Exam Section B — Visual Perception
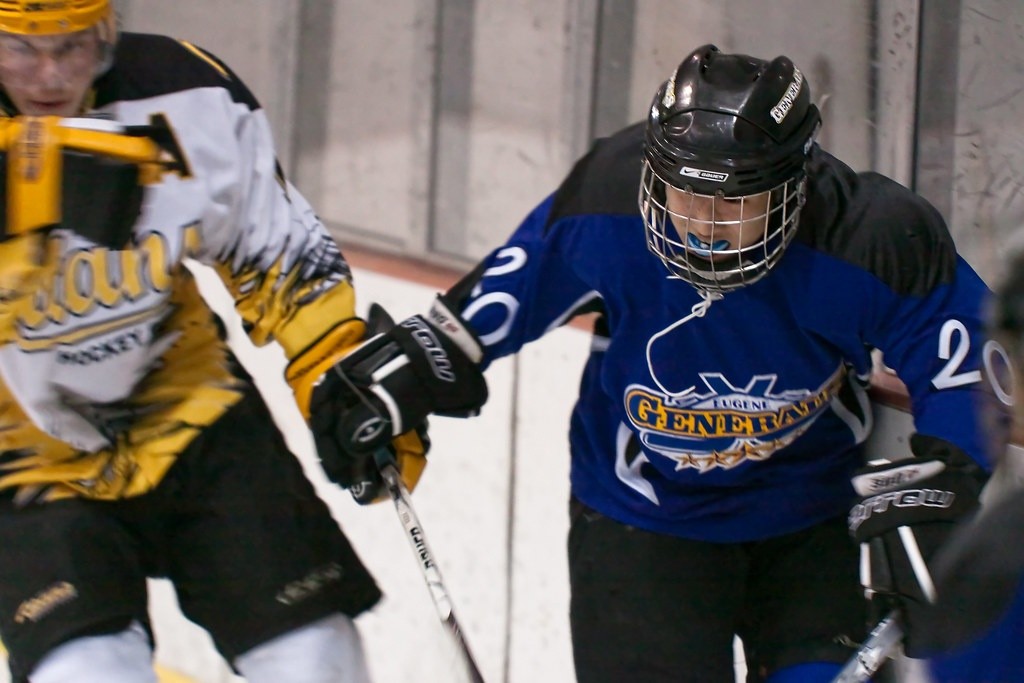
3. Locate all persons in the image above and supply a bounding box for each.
[311,43,1024,683]
[0,0,428,683]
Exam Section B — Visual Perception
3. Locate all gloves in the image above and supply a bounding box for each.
[308,292,490,506]
[282,317,428,504]
[840,452,980,661]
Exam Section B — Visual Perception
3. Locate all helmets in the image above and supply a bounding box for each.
[638,44,823,293]
[0,0,117,46]
